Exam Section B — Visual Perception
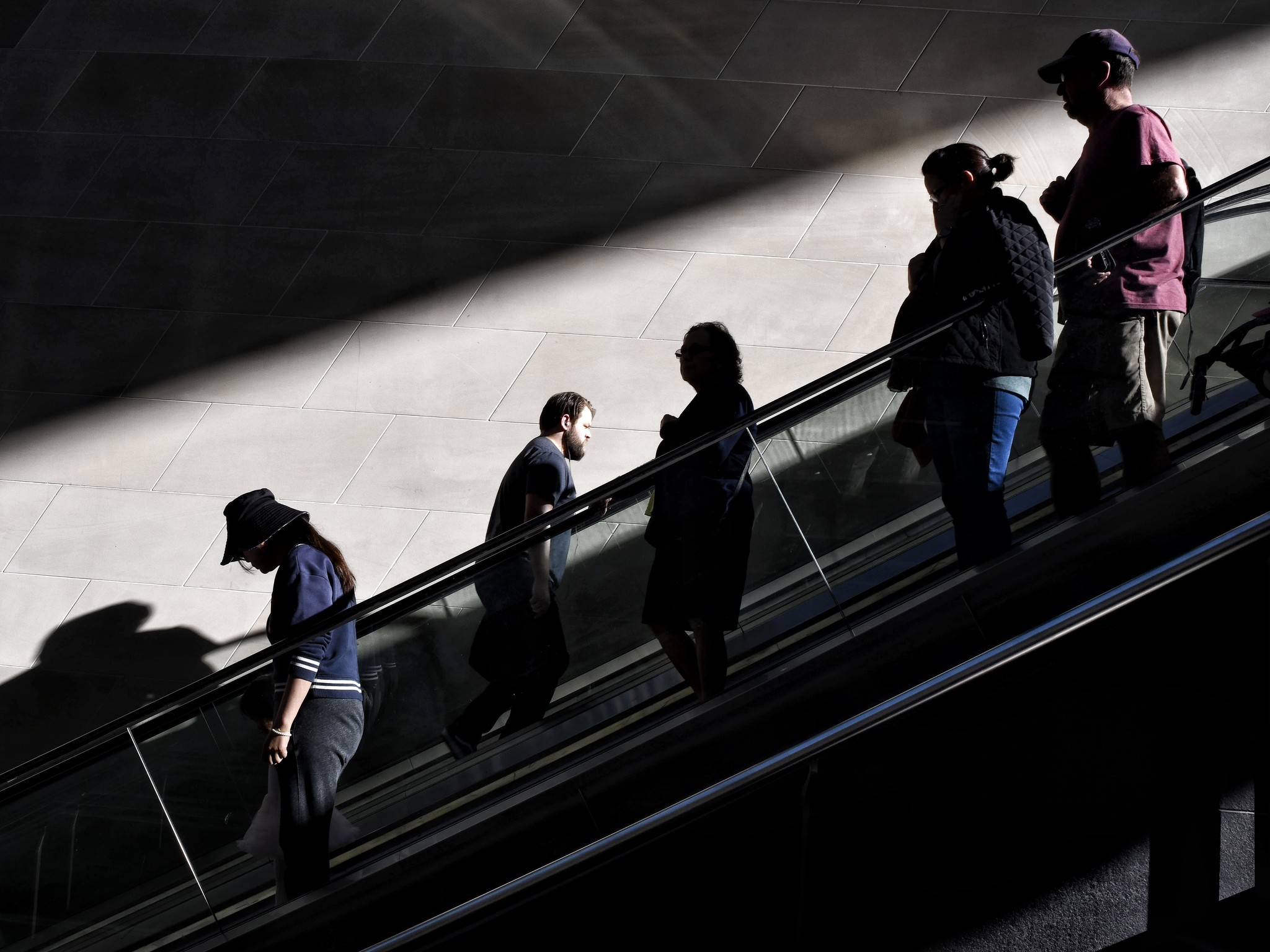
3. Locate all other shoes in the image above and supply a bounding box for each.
[441,724,477,761]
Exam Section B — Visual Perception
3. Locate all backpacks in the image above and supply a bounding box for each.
[1110,105,1204,317]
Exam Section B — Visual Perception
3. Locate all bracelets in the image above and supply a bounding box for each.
[276,727,291,733]
[270,727,292,736]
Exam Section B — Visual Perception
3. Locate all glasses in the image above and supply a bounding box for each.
[674,349,712,359]
[929,182,950,203]
[1059,69,1097,82]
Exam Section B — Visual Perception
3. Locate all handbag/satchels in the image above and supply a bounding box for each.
[236,762,358,858]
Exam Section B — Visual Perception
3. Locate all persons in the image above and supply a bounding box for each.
[641,321,757,706]
[887,144,1055,572]
[219,488,365,908]
[1038,29,1189,521]
[442,391,612,760]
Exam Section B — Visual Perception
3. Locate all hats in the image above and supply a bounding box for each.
[220,488,310,566]
[1037,29,1139,84]
